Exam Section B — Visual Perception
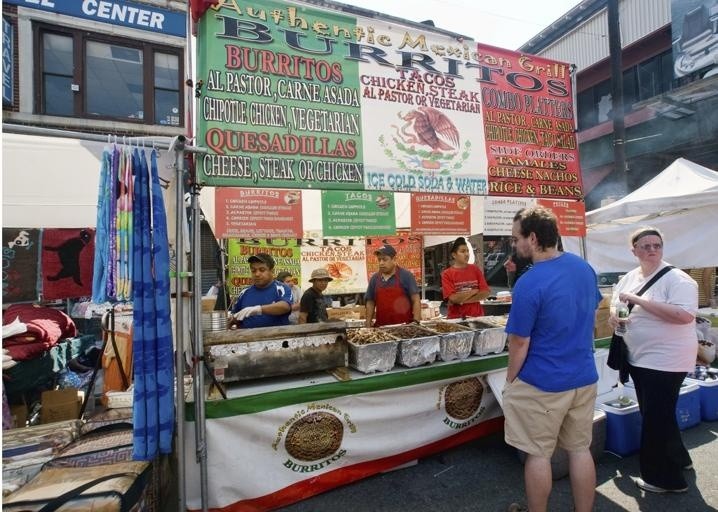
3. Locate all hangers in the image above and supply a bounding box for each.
[96,134,164,157]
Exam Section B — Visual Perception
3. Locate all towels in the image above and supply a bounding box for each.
[87,146,176,462]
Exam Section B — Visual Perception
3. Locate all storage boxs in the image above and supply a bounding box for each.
[697,369,717,423]
[624,377,703,433]
[519,407,608,479]
[597,393,645,459]
[8,393,28,428]
[41,386,84,423]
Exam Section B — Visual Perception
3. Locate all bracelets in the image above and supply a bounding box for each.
[412,320,419,325]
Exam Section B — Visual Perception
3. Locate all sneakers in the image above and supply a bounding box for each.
[636,478,688,494]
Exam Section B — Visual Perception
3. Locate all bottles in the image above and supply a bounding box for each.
[614,304,628,335]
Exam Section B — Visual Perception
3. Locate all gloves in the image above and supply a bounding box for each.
[233,305,262,321]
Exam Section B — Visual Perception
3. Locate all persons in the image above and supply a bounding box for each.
[297,269,333,323]
[363,246,421,327]
[277,272,295,291]
[440,238,491,319]
[228,252,294,329]
[502,255,517,290]
[609,225,702,495]
[501,203,602,511]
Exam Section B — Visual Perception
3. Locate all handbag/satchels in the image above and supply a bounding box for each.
[607,330,629,386]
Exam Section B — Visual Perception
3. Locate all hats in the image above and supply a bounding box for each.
[448,237,465,252]
[308,269,333,282]
[248,252,274,267]
[373,245,395,257]
